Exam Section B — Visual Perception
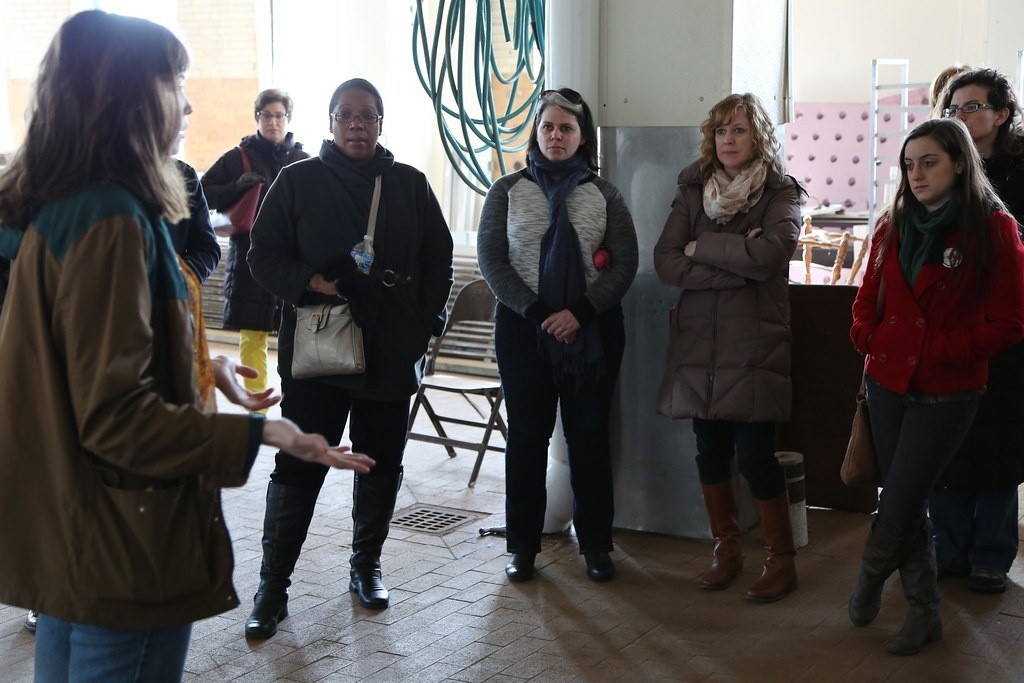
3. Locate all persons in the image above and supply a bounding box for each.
[654,94,798,600]
[246,79,454,638]
[849,119,1024,655]
[929,65,1024,594]
[478,88,639,581]
[199,90,309,415]
[0,10,376,683]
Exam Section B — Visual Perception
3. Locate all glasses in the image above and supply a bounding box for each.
[257,111,288,121]
[539,88,586,111]
[331,111,383,124]
[942,102,995,118]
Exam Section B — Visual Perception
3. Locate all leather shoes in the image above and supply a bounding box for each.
[583,552,614,580]
[506,552,536,581]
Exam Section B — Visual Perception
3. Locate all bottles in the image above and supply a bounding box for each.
[336,235,376,301]
[593,248,612,270]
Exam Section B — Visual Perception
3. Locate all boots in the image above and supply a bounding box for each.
[244,481,320,640]
[885,519,945,651]
[849,513,925,628]
[696,483,743,589]
[745,493,797,602]
[350,465,404,608]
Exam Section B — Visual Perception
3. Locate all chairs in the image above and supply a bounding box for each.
[407,279,508,491]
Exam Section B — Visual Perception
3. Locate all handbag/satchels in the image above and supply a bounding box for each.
[291,304,365,380]
[211,146,262,237]
[840,400,884,486]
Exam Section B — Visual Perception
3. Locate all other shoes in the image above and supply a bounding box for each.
[972,565,1007,592]
[936,555,958,577]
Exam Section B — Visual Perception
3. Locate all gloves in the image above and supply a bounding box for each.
[235,172,266,195]
